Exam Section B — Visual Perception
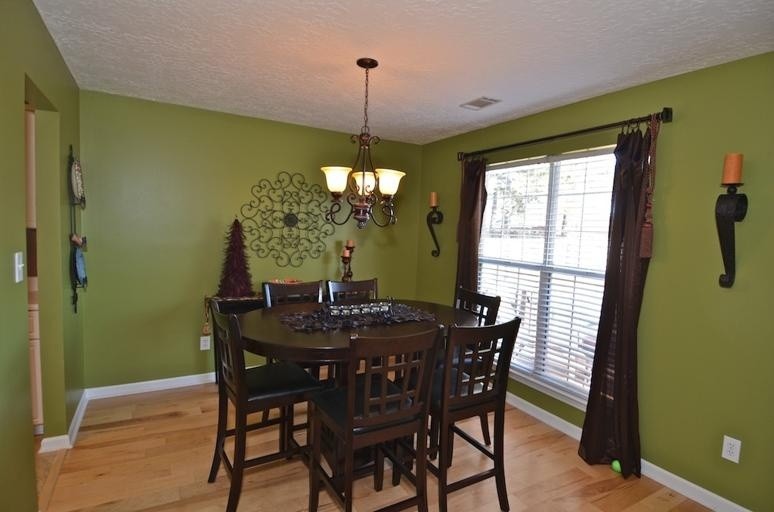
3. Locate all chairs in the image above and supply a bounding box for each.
[309,324,448,512]
[209,299,323,512]
[397,286,503,461]
[258,279,327,453]
[392,316,524,512]
[328,277,379,304]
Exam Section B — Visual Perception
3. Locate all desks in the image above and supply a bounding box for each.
[237,299,479,482]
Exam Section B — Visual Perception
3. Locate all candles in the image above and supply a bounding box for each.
[722,153,743,184]
[429,191,438,207]
[343,240,353,257]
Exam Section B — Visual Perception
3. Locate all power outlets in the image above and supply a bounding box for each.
[721,435,742,464]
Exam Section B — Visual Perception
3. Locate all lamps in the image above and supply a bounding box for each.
[320,57,407,230]
